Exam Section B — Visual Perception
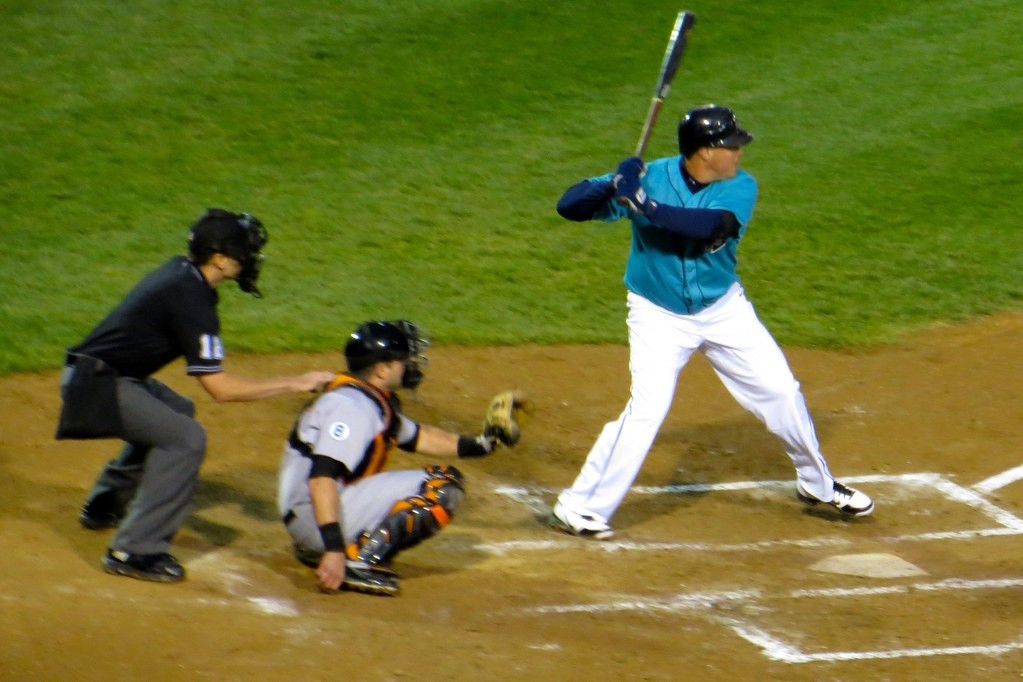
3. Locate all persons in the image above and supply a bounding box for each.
[278,320,534,596]
[549,104,875,540]
[55,207,334,584]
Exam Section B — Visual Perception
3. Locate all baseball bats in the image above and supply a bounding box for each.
[616,10,697,208]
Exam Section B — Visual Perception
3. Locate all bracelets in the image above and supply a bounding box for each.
[320,522,345,552]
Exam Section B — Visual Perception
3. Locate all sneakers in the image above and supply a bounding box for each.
[550,499,614,541]
[79,503,129,529]
[795,474,873,518]
[101,546,183,582]
[340,561,399,596]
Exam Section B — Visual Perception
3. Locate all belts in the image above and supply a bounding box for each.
[282,510,296,527]
[66,353,79,366]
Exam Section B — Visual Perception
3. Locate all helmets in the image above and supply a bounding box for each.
[188,205,267,266]
[677,103,753,159]
[344,320,408,371]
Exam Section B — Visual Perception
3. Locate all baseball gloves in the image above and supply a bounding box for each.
[482,388,535,450]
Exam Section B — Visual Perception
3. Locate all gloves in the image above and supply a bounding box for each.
[616,178,656,218]
[613,156,648,187]
